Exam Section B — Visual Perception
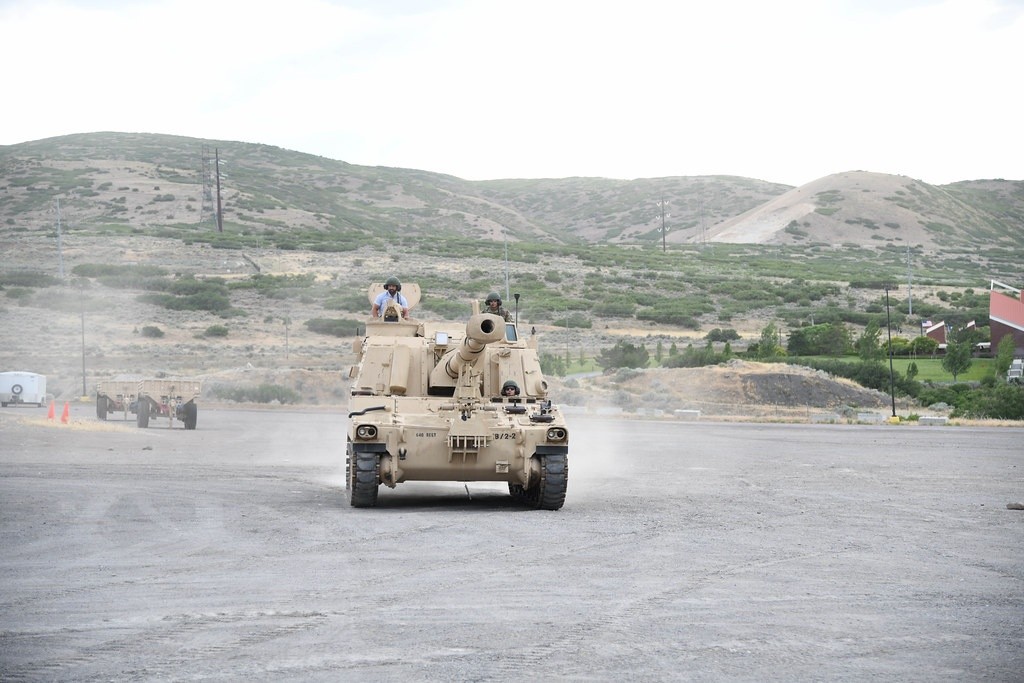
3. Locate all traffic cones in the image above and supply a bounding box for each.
[59,401,69,422]
[46,401,56,420]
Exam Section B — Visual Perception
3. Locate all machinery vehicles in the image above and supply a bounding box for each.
[347,282,570,511]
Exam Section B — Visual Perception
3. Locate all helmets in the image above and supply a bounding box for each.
[383,276,401,291]
[485,293,502,306]
[501,380,520,394]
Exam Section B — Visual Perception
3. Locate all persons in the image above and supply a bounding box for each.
[482,293,515,323]
[501,380,521,397]
[371,276,409,320]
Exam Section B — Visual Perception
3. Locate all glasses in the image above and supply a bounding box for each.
[490,300,497,302]
[506,388,514,390]
